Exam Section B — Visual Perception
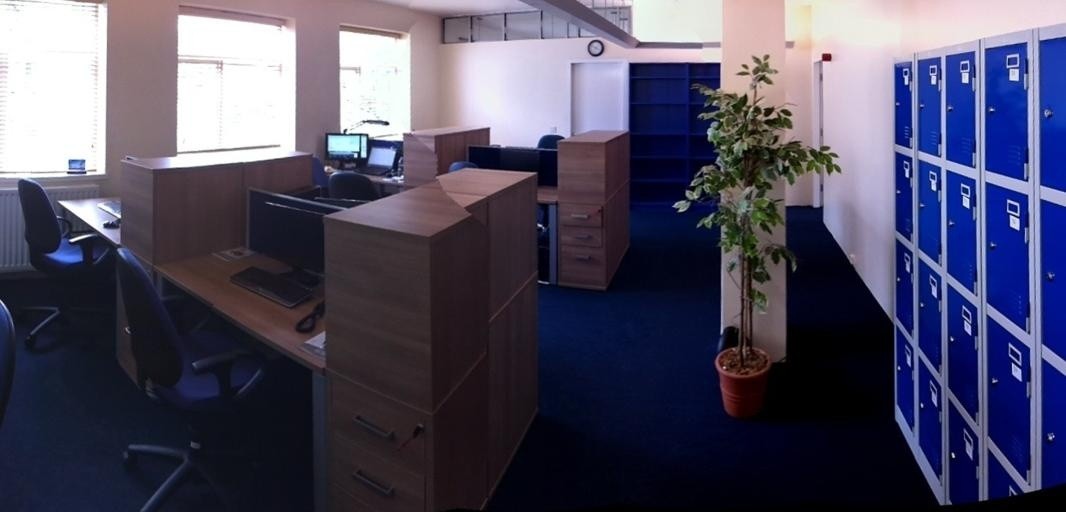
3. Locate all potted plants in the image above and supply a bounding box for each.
[672,54,843,418]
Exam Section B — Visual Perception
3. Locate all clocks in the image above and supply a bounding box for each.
[587,40,604,57]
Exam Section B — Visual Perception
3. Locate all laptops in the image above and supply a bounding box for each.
[353,147,397,175]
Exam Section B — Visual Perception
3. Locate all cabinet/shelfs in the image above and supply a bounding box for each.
[628,62,720,205]
[893,23,1066,512]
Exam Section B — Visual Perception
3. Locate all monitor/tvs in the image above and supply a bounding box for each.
[325,132,369,171]
[245,186,348,286]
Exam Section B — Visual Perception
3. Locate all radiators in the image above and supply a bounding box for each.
[0,184,101,272]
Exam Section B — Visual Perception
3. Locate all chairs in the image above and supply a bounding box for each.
[16,179,110,347]
[0,301,17,416]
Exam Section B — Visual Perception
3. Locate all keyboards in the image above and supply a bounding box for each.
[98,199,121,219]
[231,267,312,307]
